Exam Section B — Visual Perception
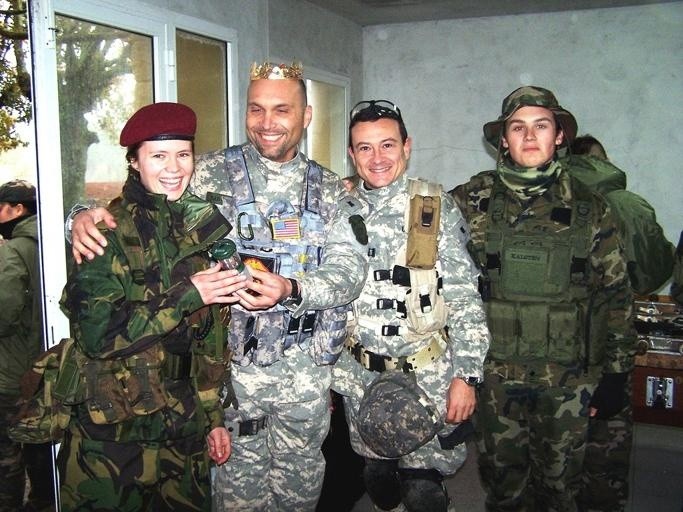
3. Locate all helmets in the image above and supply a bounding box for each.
[357,370,444,458]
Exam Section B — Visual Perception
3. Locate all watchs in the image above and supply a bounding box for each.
[280,277,299,307]
[461,376,481,385]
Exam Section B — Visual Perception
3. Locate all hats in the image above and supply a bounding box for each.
[0,180,35,196]
[483,86,577,152]
[120,102,196,147]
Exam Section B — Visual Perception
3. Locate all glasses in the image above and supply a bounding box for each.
[349,100,401,122]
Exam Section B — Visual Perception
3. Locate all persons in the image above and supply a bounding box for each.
[445,87,638,512]
[64,67,370,511]
[328,107,495,512]
[56,102,232,512]
[560,134,677,512]
[0,179,58,512]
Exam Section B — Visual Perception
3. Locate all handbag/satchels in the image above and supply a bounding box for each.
[8,337,78,445]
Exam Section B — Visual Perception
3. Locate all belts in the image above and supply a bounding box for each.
[345,331,449,373]
[287,313,317,335]
